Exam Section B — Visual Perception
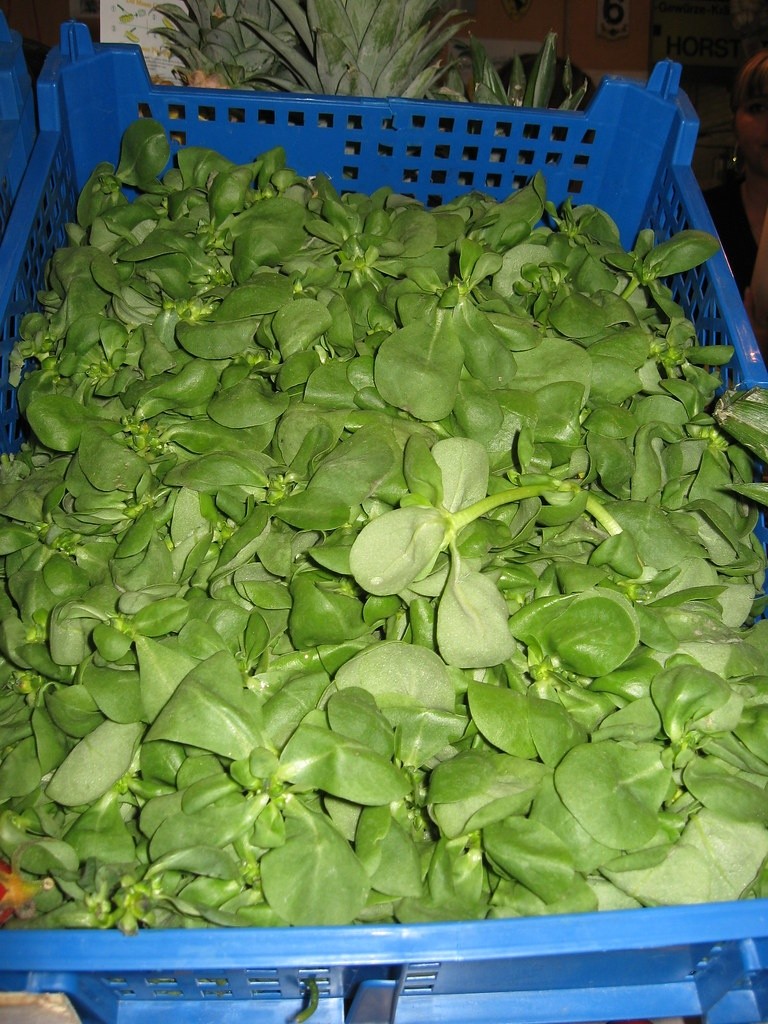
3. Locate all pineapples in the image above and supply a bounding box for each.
[146,0,311,145]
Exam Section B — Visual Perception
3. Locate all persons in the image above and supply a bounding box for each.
[702,46,768,363]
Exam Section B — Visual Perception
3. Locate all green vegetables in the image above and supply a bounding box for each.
[0,109,767,934]
